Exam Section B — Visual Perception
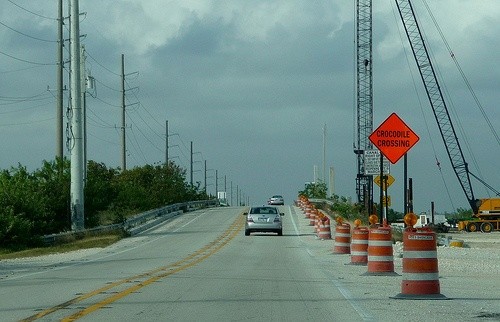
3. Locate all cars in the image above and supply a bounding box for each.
[267,198,271,205]
[243,205,285,236]
[269,194,284,205]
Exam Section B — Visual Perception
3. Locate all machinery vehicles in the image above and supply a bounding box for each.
[457,198,500,233]
